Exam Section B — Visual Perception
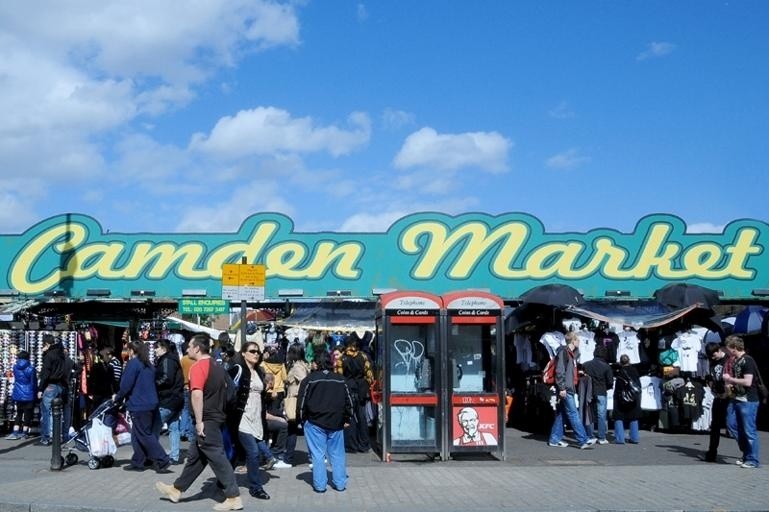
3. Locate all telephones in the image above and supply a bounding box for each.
[416,357,432,389]
[449,358,463,388]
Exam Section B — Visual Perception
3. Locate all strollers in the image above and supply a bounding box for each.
[51,395,126,470]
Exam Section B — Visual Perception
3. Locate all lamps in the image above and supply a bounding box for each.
[716,290,725,297]
[577,289,585,297]
[278,289,304,297]
[1,290,20,297]
[87,289,111,299]
[131,290,156,297]
[327,290,352,296]
[182,289,207,297]
[373,289,397,296]
[45,288,70,296]
[751,290,769,297]
[606,290,631,296]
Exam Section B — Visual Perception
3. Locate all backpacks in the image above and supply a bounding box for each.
[543,360,555,384]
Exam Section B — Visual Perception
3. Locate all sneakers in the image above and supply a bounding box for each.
[736,459,754,468]
[255,489,270,501]
[239,456,293,472]
[549,438,608,449]
[157,458,178,473]
[155,482,181,502]
[34,442,48,446]
[5,433,18,440]
[213,496,243,511]
[124,465,144,471]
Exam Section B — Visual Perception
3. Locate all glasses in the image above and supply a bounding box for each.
[248,350,261,354]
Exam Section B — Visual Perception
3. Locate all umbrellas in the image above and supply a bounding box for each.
[653,282,719,322]
[731,305,769,336]
[520,283,586,324]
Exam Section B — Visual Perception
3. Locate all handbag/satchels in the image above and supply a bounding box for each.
[622,379,640,403]
